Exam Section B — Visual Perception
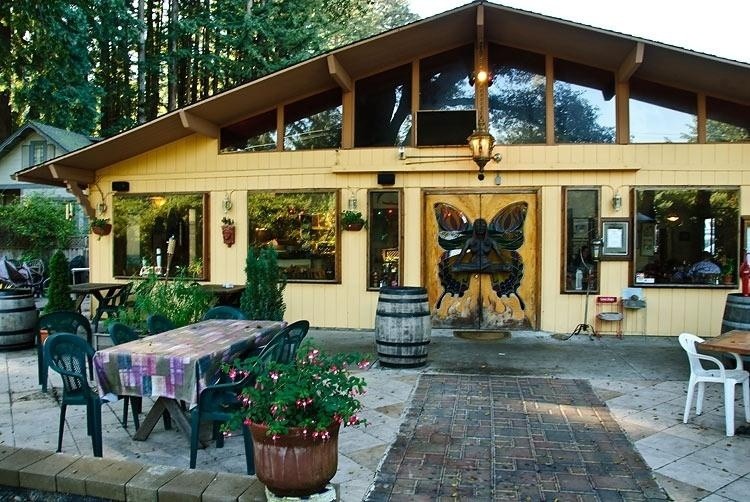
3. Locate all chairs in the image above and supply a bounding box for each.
[185,320,309,476]
[679,332,750,437]
[44,305,245,460]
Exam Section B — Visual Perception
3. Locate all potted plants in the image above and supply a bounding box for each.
[91,217,112,235]
[339,210,365,231]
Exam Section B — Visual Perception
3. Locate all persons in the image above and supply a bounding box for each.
[451,218,514,275]
[685,251,723,280]
[574,245,595,283]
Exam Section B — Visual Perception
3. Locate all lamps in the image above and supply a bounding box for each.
[613,189,622,212]
[466,115,502,173]
[348,192,357,210]
[222,191,232,215]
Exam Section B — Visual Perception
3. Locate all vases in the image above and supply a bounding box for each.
[248,424,340,498]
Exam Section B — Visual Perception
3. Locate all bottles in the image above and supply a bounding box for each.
[576,267,582,290]
[715,276,719,285]
[739,253,750,297]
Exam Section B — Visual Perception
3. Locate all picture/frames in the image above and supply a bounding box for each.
[600,217,634,261]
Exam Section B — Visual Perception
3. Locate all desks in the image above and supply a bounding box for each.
[67,280,245,330]
[697,330,750,355]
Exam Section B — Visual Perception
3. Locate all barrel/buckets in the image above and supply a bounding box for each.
[721,293,750,384]
[0,288,37,351]
[375,286,432,368]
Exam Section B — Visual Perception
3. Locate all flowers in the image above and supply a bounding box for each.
[219,343,371,440]
